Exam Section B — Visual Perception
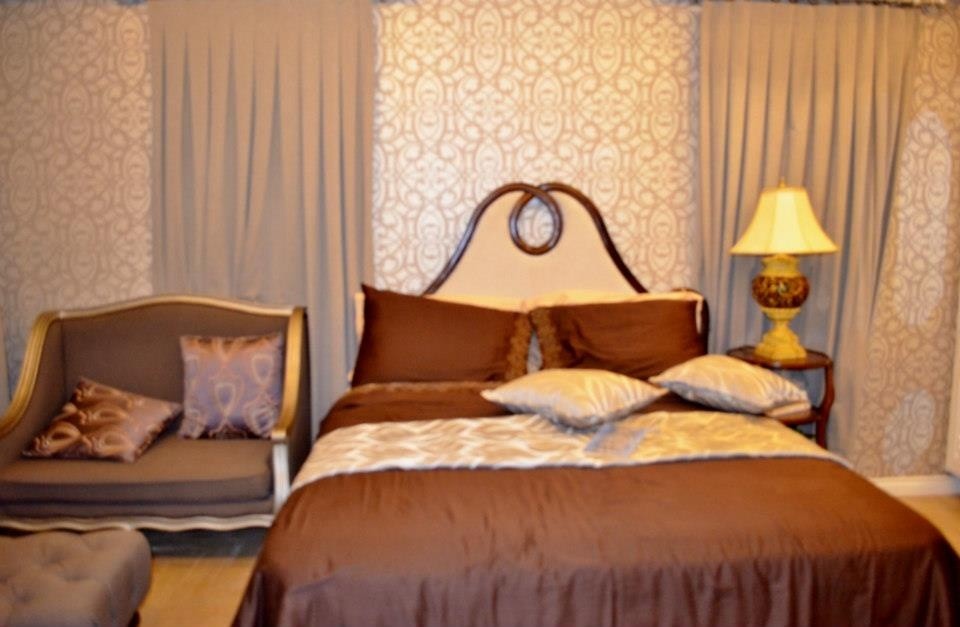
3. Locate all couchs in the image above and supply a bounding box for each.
[0,532,154,627]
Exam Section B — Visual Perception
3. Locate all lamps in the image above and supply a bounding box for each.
[729,175,839,359]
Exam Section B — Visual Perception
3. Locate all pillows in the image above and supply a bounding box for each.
[480,368,670,429]
[179,332,283,441]
[649,353,809,414]
[24,376,182,466]
[530,288,704,383]
[348,281,533,387]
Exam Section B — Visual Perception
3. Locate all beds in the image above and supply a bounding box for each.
[257,181,960,627]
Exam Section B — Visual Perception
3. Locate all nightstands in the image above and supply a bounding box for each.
[726,344,835,453]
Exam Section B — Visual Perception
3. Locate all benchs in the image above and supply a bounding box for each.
[0,297,315,532]
